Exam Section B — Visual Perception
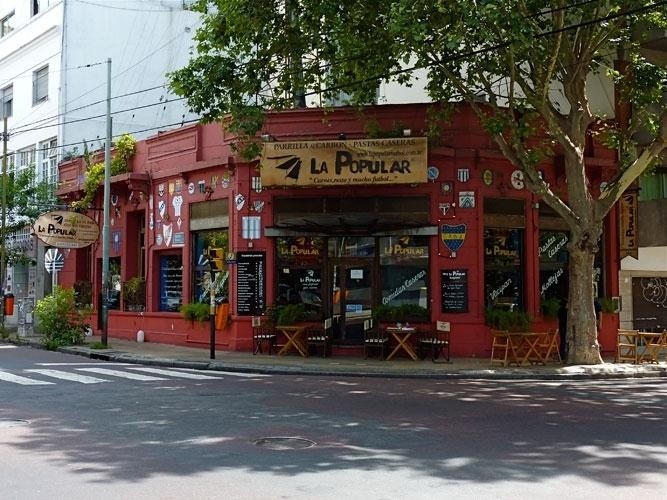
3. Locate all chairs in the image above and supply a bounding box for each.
[250,313,452,364]
[489,327,666,368]
